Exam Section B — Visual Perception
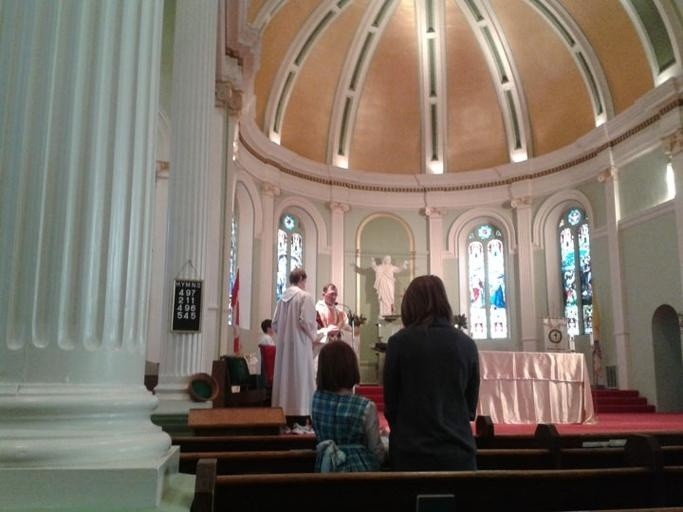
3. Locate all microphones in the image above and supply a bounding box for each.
[334,302,351,312]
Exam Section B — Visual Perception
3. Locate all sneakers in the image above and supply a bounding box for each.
[286,422,316,435]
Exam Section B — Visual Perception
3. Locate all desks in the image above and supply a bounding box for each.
[478,350,585,424]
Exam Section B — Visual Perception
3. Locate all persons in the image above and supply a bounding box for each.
[311,340,385,473]
[383,275,480,472]
[259,319,275,346]
[312,283,353,383]
[271,268,317,435]
[371,256,407,318]
